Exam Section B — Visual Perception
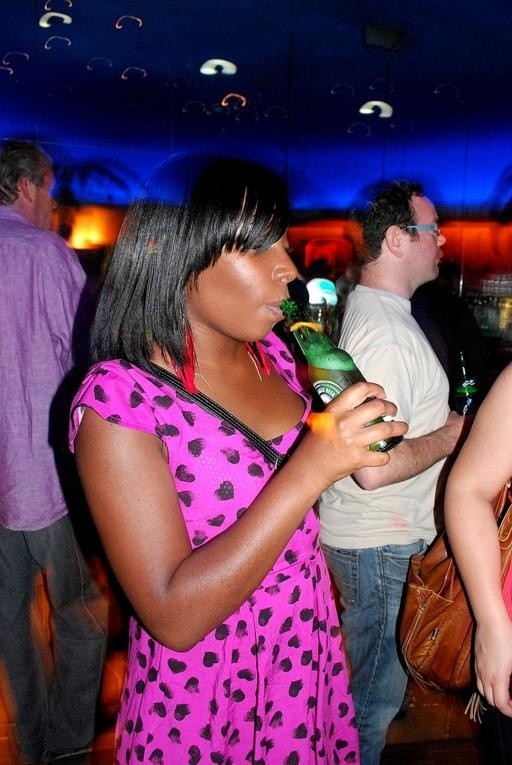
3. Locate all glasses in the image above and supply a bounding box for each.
[400,223,440,237]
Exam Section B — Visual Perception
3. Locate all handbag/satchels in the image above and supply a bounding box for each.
[396,478,512,697]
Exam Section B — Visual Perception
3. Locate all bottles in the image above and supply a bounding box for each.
[279,295,405,454]
[450,347,480,418]
